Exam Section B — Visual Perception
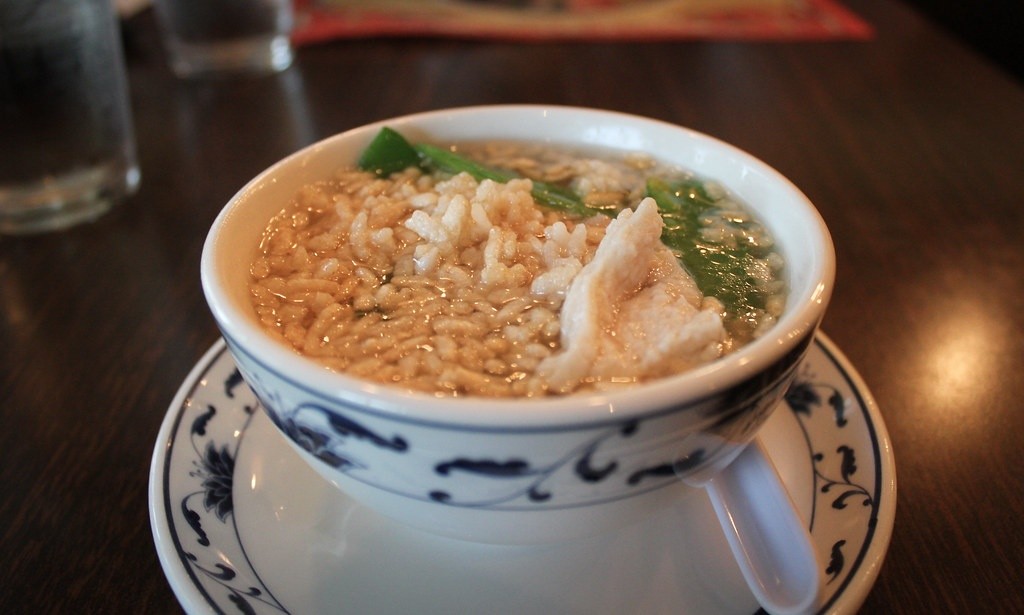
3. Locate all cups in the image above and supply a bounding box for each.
[0,0,146,237]
[154,0,294,79]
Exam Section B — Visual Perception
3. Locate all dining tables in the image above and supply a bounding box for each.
[0,0,1024,615]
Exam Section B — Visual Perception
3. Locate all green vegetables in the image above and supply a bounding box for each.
[355,124,785,322]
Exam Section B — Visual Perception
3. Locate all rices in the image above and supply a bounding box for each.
[250,141,783,399]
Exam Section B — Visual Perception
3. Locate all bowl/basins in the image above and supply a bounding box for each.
[200,104,836,546]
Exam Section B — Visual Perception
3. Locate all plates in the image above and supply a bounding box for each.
[148,324,898,615]
